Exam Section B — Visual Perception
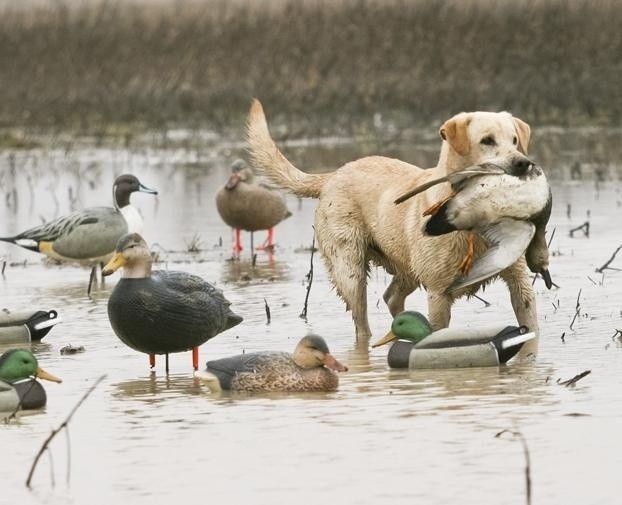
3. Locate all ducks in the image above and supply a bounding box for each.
[101,232,244,369]
[216,160,293,248]
[393,162,552,289]
[0,173,159,284]
[194,333,348,393]
[0,348,63,412]
[0,307,64,345]
[371,310,537,369]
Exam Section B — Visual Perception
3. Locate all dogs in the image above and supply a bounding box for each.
[242,97,540,339]
